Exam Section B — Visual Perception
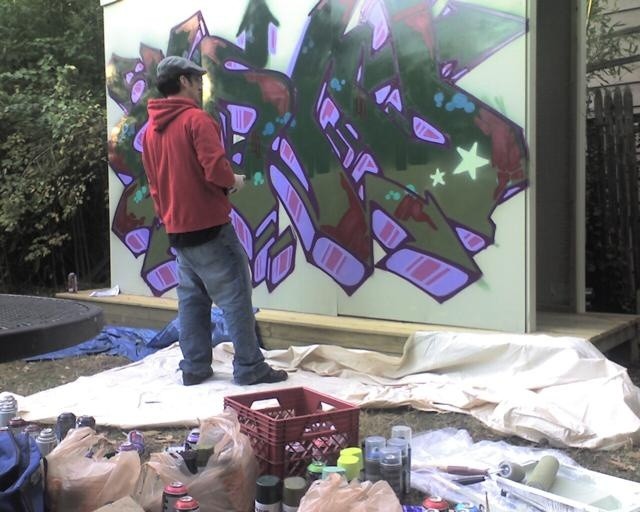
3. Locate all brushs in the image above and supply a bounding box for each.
[411,459,525,482]
[452,455,559,492]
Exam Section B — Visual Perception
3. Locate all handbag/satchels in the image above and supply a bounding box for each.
[0,428,46,512]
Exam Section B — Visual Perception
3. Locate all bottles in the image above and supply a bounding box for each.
[422,494,480,512]
[0,392,145,456]
[173,495,200,512]
[254,424,411,512]
[68,273,78,292]
[184,428,200,453]
[161,481,188,512]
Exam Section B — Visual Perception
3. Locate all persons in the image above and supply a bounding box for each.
[138,55,290,385]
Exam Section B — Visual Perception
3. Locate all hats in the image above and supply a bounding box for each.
[156,56,208,77]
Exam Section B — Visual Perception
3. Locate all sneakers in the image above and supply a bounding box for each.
[183,366,213,385]
[236,365,288,385]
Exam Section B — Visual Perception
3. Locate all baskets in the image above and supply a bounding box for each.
[224,386,360,476]
